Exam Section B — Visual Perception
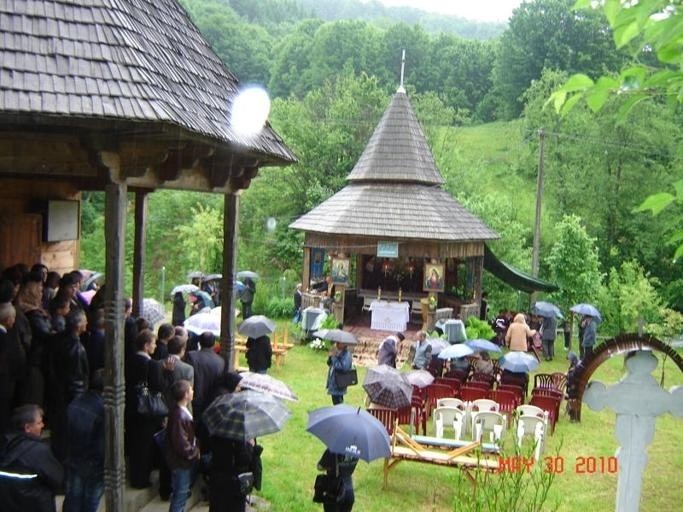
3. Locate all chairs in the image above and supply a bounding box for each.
[514,414,547,462]
[395,401,428,437]
[365,406,401,439]
[413,355,569,431]
[361,296,377,312]
[409,300,423,318]
[432,405,467,440]
[470,410,507,443]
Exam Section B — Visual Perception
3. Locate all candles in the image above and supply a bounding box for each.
[377,284,381,297]
[398,286,401,298]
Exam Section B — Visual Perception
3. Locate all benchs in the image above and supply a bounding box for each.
[377,435,513,491]
[232,329,293,372]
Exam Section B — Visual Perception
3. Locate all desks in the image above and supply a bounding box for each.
[369,300,409,332]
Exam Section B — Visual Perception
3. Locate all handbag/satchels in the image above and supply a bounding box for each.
[137,388,170,417]
[154,428,172,456]
[335,369,357,389]
[313,474,346,505]
[234,472,254,497]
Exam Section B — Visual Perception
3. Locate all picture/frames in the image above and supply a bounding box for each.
[422,263,445,293]
[330,254,351,286]
